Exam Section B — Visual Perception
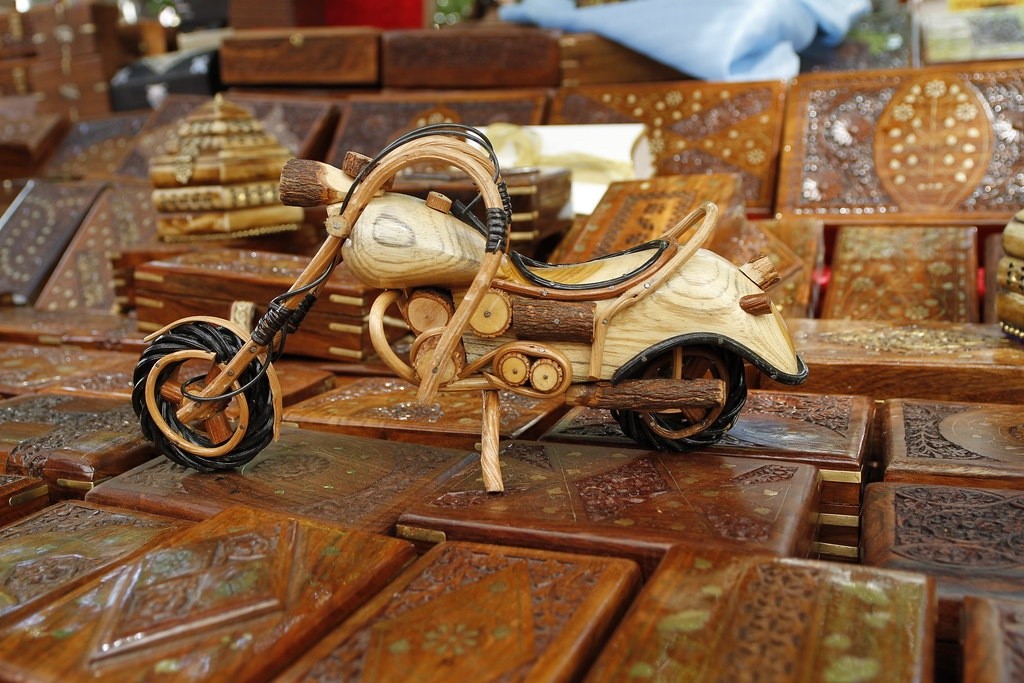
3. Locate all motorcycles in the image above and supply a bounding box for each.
[129,120,811,501]
